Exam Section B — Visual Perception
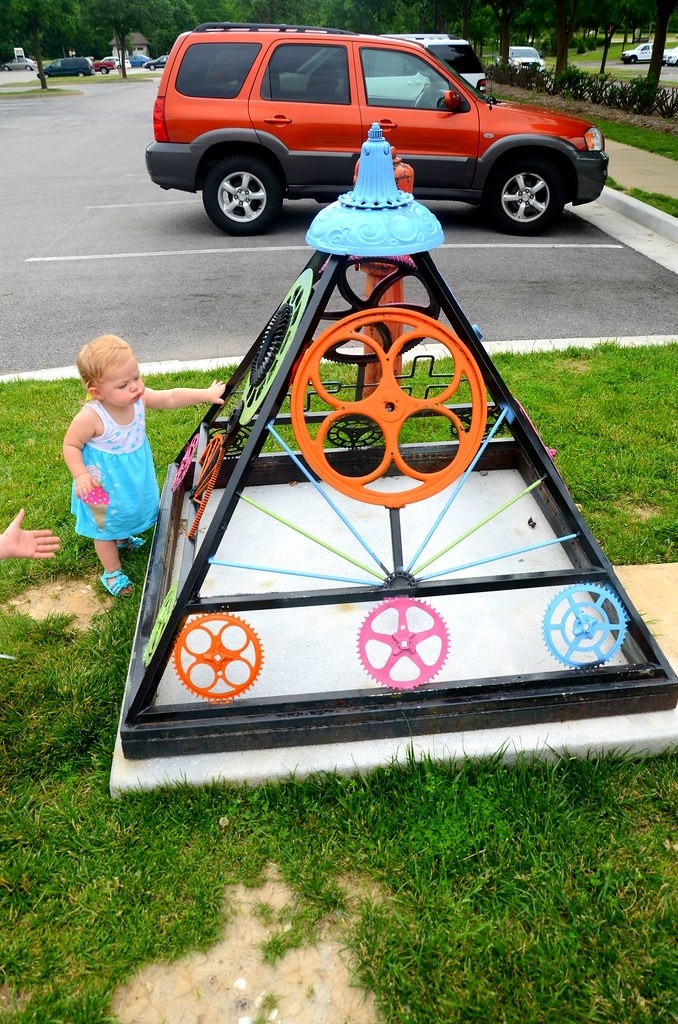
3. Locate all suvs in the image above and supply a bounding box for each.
[229,33,486,109]
[145,23,609,236]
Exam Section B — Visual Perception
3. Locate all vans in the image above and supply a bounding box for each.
[37,58,96,78]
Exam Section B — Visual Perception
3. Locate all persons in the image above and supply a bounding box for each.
[62,333,226,596]
[0,507,61,560]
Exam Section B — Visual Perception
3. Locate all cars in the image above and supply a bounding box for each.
[101,56,132,71]
[496,46,546,75]
[0,58,38,71]
[666,47,678,66]
[144,55,169,71]
[126,55,152,67]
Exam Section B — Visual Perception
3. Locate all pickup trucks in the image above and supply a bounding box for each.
[621,43,673,64]
[88,57,116,74]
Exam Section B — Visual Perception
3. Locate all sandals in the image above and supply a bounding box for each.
[117,536,144,549]
[101,568,134,597]
